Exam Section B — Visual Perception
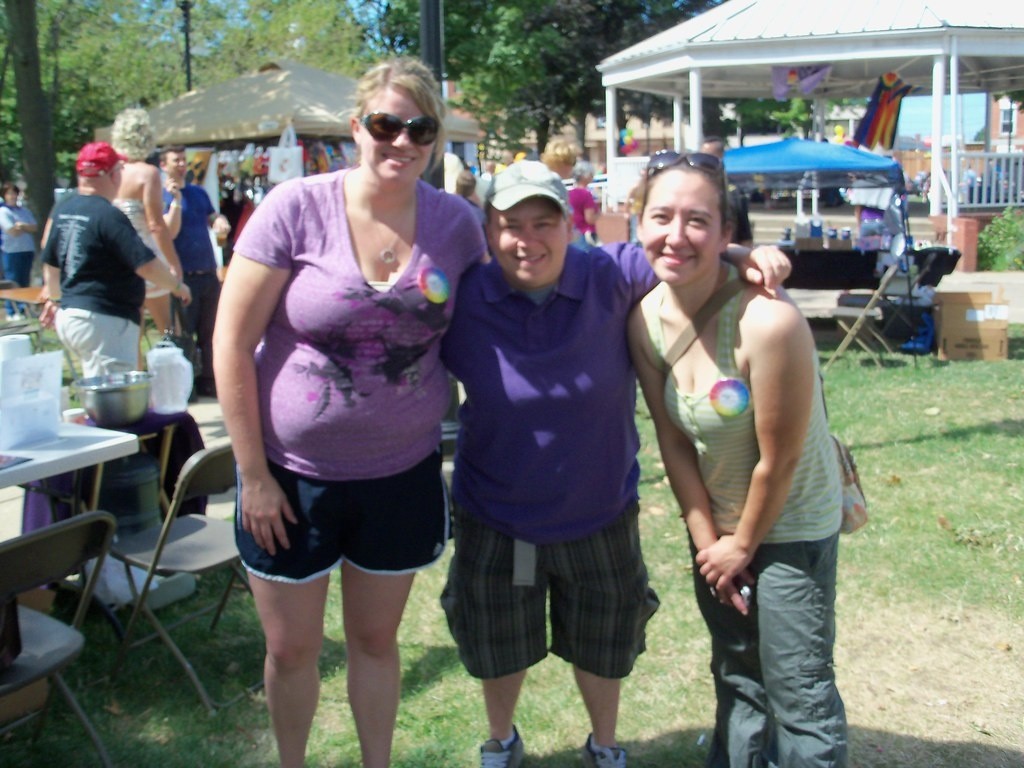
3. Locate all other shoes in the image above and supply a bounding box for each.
[6,313,27,323]
[197,376,217,397]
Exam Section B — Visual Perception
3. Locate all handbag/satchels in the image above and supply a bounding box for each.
[832,432,870,539]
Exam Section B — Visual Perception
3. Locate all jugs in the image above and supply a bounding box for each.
[146,342,193,414]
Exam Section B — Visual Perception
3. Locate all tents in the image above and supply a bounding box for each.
[93,56,480,151]
[719,137,919,371]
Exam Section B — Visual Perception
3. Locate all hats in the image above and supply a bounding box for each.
[77,141,129,178]
[484,158,572,220]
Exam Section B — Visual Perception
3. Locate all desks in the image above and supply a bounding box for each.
[0,428,137,641]
[776,241,889,327]
[56,409,201,584]
[0,286,82,379]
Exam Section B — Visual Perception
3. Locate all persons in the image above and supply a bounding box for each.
[439,159,792,768]
[626,149,847,768]
[702,136,752,248]
[211,54,491,768]
[38,140,191,381]
[455,136,602,245]
[0,104,231,370]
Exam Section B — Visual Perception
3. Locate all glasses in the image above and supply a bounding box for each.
[643,148,729,214]
[356,110,439,148]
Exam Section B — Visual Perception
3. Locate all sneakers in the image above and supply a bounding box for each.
[582,731,628,768]
[480,723,524,768]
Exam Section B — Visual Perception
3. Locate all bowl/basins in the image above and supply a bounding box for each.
[70,371,157,428]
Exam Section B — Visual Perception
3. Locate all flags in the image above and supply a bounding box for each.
[771,63,833,101]
[853,72,923,150]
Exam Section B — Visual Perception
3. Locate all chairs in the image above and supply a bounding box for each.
[111,441,267,718]
[786,252,936,376]
[0,510,116,768]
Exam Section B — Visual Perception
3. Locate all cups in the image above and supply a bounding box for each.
[217,228,227,246]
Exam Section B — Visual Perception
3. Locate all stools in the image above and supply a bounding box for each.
[0,320,44,353]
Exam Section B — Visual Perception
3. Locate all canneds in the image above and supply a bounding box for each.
[828,228,851,240]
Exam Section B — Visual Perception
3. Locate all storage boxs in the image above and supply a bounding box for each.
[933,287,1008,360]
[1,590,56,722]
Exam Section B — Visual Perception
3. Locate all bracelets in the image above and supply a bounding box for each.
[172,280,182,293]
[171,202,182,209]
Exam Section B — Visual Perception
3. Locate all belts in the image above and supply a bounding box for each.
[179,265,218,276]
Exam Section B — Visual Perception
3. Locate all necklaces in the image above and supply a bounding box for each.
[348,165,417,264]
